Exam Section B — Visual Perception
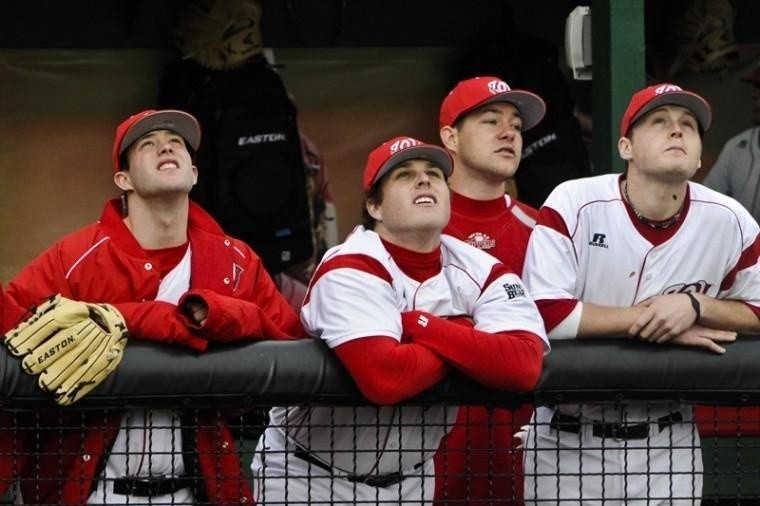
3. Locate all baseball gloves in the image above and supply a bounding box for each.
[5,293,130,411]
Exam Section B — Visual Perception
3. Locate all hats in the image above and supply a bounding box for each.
[439,76,546,131]
[362,136,454,191]
[621,83,712,137]
[112,110,201,172]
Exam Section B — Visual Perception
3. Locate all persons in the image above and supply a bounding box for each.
[416,74,548,505]
[9,107,300,504]
[700,119,758,228]
[0,287,42,504]
[523,80,757,504]
[250,135,544,505]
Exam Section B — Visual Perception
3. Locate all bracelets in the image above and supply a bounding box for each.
[685,288,704,327]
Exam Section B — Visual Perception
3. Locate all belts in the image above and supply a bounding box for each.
[94,478,189,497]
[295,445,426,488]
[550,411,681,442]
